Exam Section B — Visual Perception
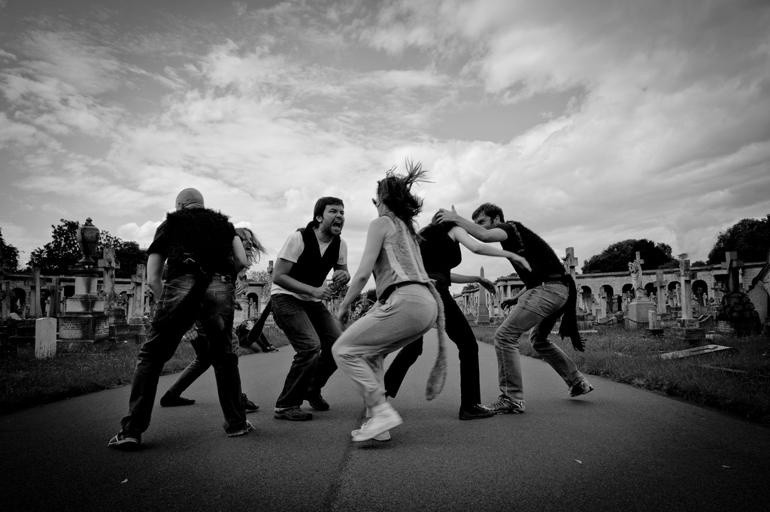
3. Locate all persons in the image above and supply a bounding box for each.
[270,196,351,421]
[236,321,280,353]
[436,203,594,415]
[160,227,269,411]
[106,188,252,447]
[331,158,438,442]
[383,211,533,420]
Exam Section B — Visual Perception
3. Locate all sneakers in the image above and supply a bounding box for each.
[350,402,405,443]
[458,400,525,420]
[240,391,259,411]
[307,390,330,411]
[568,377,595,397]
[160,395,196,406]
[107,429,142,447]
[224,420,253,437]
[273,405,312,421]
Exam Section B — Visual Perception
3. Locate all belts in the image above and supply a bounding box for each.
[379,281,429,305]
